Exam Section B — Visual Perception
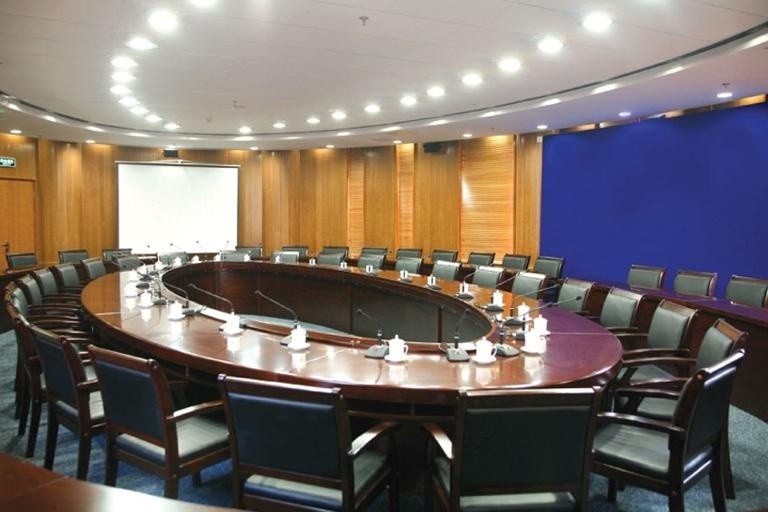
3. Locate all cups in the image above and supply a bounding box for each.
[226,315,240,331]
[140,290,151,305]
[189,253,250,264]
[123,256,182,291]
[384,333,410,359]
[309,258,411,281]
[427,274,436,285]
[170,301,182,317]
[457,281,553,364]
[290,323,309,346]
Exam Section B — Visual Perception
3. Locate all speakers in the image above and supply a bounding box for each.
[423,142,442,153]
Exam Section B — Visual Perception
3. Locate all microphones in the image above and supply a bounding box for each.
[132,251,195,317]
[143,240,230,261]
[188,282,245,336]
[425,261,582,362]
[356,308,395,364]
[253,290,309,349]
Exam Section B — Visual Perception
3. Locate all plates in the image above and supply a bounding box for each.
[223,327,244,336]
[124,292,138,296]
[137,303,154,308]
[383,354,410,362]
[424,284,440,288]
[167,314,185,321]
[286,341,311,350]
